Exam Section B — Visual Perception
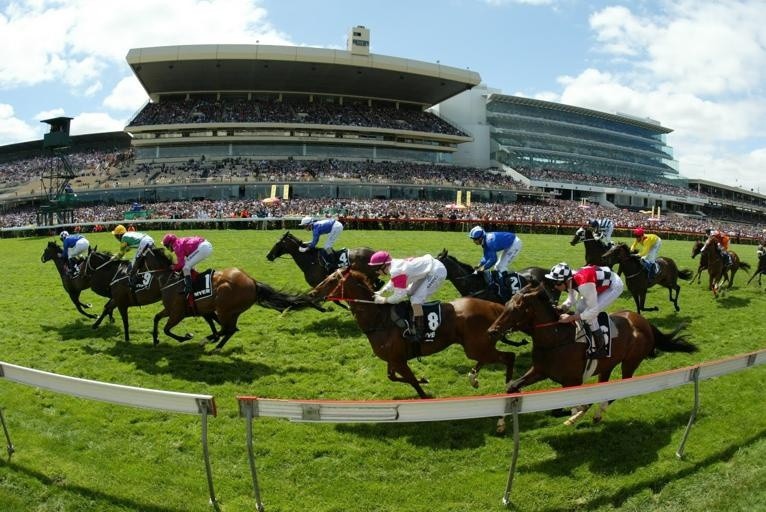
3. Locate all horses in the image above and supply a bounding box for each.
[434,247,561,308]
[265,231,386,311]
[79,245,161,344]
[569,224,631,277]
[41,240,99,320]
[129,243,326,354]
[486,275,703,433]
[689,239,751,285]
[700,238,740,296]
[602,240,693,315]
[307,261,516,400]
[744,242,766,287]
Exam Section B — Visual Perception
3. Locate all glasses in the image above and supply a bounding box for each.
[371,267,384,271]
[552,280,563,285]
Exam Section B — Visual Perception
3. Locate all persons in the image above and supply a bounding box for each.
[542,261,626,357]
[1,148,532,195]
[589,217,614,252]
[700,225,734,260]
[507,163,708,200]
[364,250,448,342]
[108,223,154,282]
[58,229,89,276]
[128,94,469,137]
[299,215,343,267]
[630,226,663,277]
[468,224,521,295]
[755,245,766,258]
[160,232,214,295]
[1,194,765,243]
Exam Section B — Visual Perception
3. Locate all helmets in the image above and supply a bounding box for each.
[298,217,313,225]
[163,235,176,244]
[545,263,573,281]
[633,228,644,235]
[60,231,69,238]
[368,252,392,266]
[470,226,485,238]
[112,225,126,235]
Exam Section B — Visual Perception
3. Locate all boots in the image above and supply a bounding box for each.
[400,316,424,349]
[587,329,606,358]
[183,279,192,298]
